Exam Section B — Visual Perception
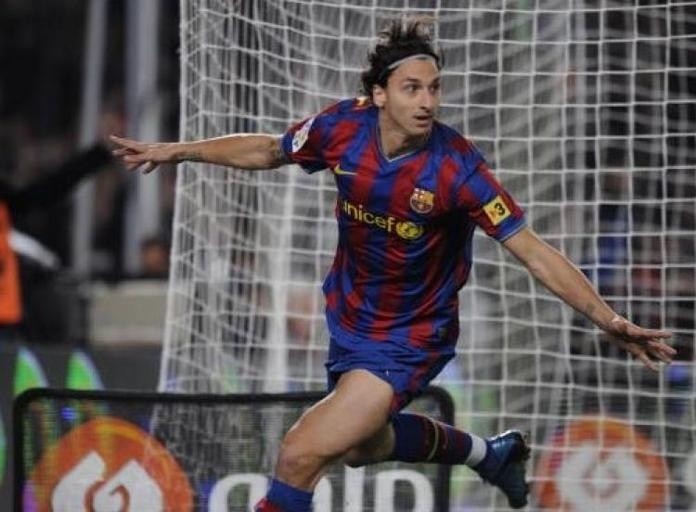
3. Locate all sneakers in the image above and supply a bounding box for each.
[484,430,531,508]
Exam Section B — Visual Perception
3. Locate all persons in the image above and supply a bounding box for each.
[111,22,676,512]
[1,108,126,418]
[124,232,174,283]
